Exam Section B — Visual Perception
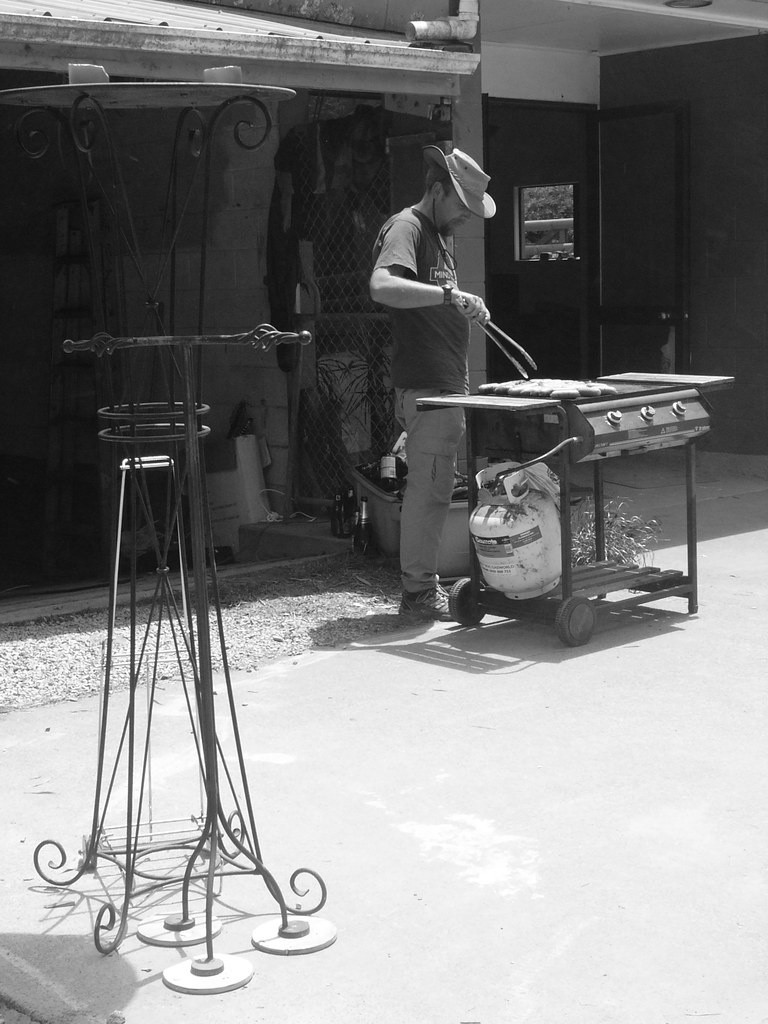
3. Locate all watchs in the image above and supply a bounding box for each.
[442,283,453,306]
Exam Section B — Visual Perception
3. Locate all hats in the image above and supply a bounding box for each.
[421,145,497,219]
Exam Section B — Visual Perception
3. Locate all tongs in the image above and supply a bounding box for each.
[454,296,538,380]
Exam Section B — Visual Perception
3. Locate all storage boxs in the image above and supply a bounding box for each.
[178,435,272,563]
[353,461,593,578]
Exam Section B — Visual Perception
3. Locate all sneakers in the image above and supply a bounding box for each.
[399,584,455,622]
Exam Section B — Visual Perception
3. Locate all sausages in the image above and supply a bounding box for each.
[479,379,619,398]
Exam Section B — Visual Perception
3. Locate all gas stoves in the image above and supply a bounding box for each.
[465,382,715,464]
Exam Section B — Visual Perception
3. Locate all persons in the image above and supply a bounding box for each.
[367,143,498,620]
